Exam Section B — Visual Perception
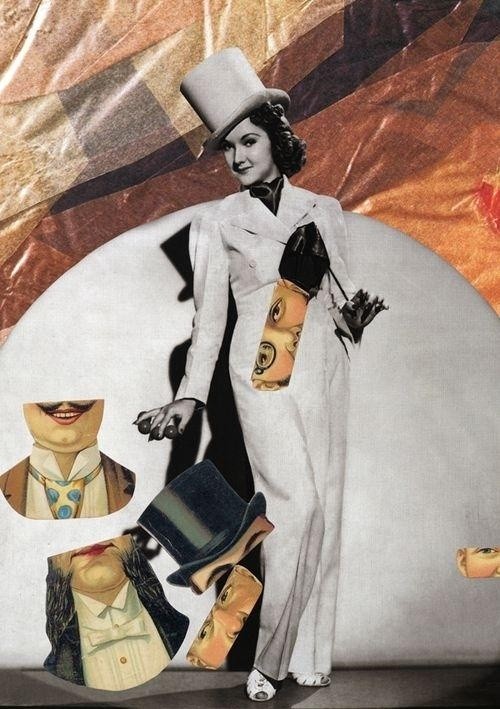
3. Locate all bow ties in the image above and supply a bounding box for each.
[78,610,150,654]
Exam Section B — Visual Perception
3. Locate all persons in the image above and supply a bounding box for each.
[456,546,500,578]
[187,566,261,670]
[42,534,189,691]
[251,283,310,393]
[1,399,136,521]
[133,47,390,700]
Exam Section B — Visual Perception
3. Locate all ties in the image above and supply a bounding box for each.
[27,461,103,518]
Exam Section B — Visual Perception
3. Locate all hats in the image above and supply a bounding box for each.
[180,47,291,164]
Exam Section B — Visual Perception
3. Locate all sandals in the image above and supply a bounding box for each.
[246,667,277,702]
[292,673,331,686]
